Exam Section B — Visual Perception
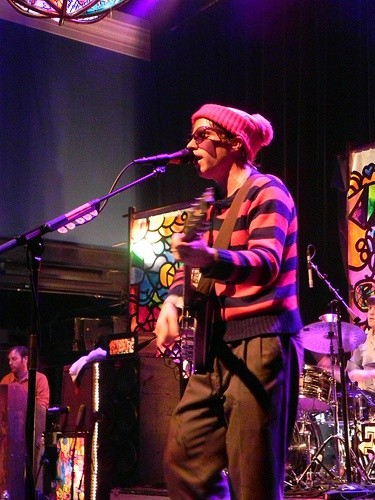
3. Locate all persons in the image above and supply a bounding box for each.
[0,345,49,500]
[153,104,301,500]
[318,295,375,393]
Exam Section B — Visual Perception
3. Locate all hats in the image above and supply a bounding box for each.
[191,104,274,163]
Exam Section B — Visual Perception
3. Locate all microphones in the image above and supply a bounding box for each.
[47,406,70,414]
[307,247,314,287]
[133,148,193,166]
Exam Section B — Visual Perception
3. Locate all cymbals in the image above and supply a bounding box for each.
[299,321,367,354]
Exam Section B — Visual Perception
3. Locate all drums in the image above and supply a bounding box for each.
[294,366,334,414]
[318,389,375,427]
[279,415,341,491]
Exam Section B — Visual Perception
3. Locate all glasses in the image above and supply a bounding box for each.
[192,126,224,145]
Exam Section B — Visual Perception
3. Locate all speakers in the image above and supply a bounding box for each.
[60,362,143,500]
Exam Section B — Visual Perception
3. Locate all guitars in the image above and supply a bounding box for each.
[178,188,214,371]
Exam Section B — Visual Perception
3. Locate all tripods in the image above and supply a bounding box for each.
[285,330,373,490]
